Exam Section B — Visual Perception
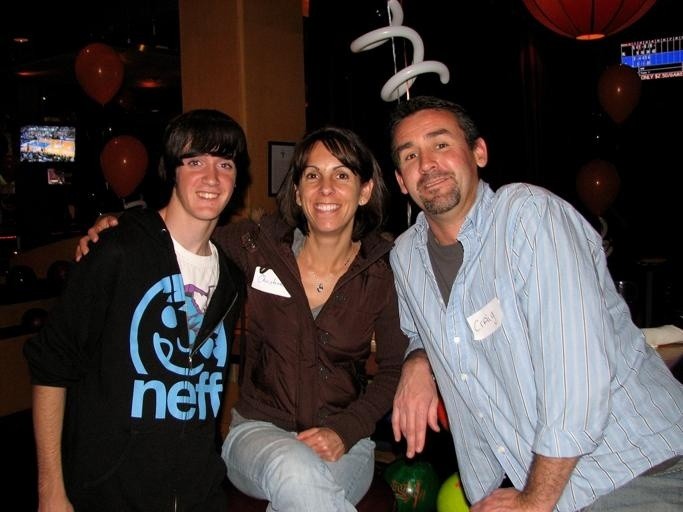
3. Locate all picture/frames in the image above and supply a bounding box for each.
[268,141,296,197]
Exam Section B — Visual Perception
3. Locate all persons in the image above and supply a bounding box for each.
[73,126,405,512]
[391,96,682,512]
[26,108,248,512]
[21,127,75,162]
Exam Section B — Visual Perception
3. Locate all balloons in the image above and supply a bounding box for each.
[75,43,122,107]
[100,136,146,199]
[575,160,619,218]
[596,64,642,123]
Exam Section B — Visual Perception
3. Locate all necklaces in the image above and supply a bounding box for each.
[302,235,354,293]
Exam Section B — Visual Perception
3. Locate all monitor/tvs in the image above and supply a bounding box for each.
[620,35,683,80]
[20,125,76,162]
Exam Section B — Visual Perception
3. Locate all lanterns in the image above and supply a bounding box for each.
[523,0,656,41]
